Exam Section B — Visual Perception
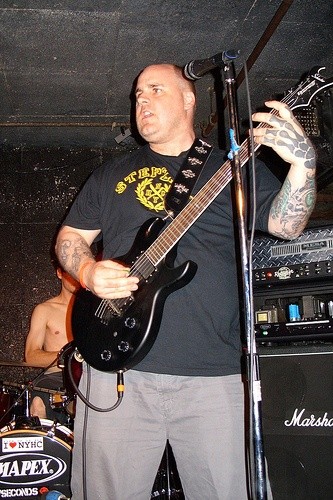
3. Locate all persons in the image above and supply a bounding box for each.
[55,64,317,500]
[25,266,83,419]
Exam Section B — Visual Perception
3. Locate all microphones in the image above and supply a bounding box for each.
[184,49,240,81]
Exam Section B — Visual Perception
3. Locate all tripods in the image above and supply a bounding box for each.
[151,445,184,500]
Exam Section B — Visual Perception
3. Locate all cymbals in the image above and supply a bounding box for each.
[1,358,46,369]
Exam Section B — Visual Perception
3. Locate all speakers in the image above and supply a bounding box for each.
[255,344,333,500]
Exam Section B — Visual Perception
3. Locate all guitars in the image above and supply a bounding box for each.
[69,61,333,375]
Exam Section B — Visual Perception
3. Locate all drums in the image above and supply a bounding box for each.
[0,418,74,500]
